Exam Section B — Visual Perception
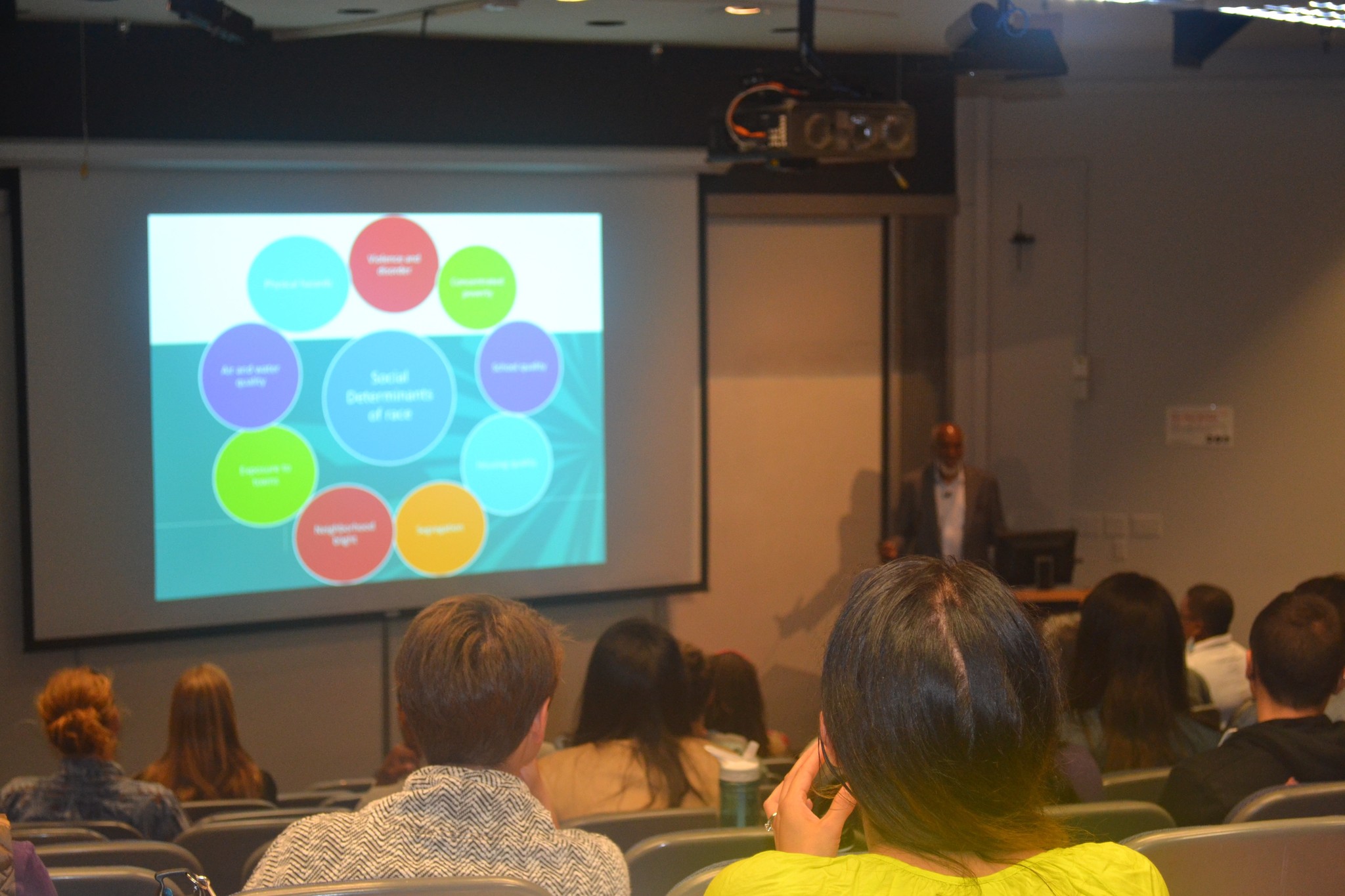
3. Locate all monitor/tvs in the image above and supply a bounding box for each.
[992,528,1080,589]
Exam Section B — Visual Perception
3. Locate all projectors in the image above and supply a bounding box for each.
[730,99,916,162]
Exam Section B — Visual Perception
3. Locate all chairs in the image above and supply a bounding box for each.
[9,691,1345,896]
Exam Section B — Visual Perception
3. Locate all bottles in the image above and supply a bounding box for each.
[704,741,762,827]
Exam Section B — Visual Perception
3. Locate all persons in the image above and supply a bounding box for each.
[244,596,630,895]
[134,663,277,804]
[376,744,420,786]
[704,554,1169,896]
[1178,584,1251,718]
[0,668,188,840]
[541,619,720,819]
[0,813,58,896]
[1042,573,1215,774]
[679,647,791,782]
[883,424,1003,562]
[1158,591,1345,826]
[1242,574,1345,726]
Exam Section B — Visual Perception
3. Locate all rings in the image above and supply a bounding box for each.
[765,812,777,831]
